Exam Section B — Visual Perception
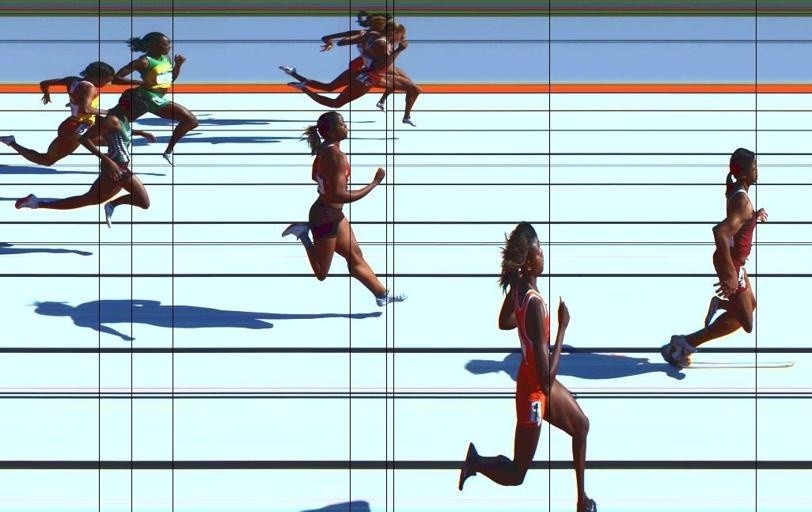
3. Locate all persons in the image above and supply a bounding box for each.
[0,61,116,166]
[278,8,422,127]
[281,110,412,308]
[110,32,201,167]
[15,88,158,229]
[656,148,770,369]
[458,220,598,512]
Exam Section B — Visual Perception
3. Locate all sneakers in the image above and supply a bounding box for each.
[279,65,296,74]
[376,102,386,113]
[287,80,306,88]
[660,335,696,368]
[0,136,15,146]
[162,150,175,166]
[15,194,37,208]
[105,201,115,228]
[376,289,408,306]
[403,118,416,126]
[282,223,311,239]
[459,442,478,490]
[577,496,597,511]
